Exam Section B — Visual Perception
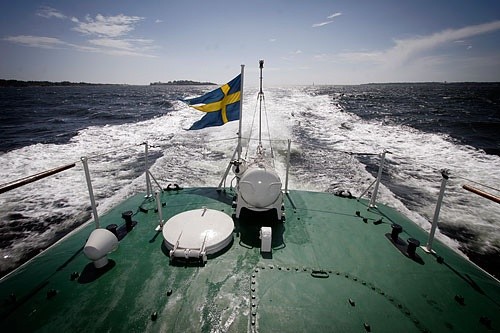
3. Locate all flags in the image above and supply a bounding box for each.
[176,73,242,131]
